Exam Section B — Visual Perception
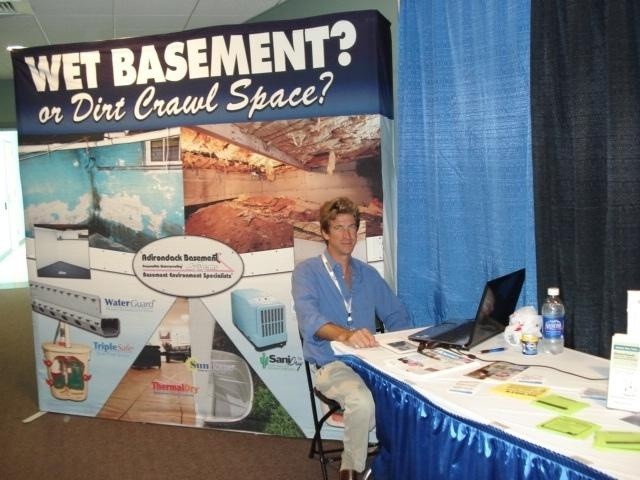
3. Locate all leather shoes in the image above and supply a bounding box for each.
[338,467,359,480]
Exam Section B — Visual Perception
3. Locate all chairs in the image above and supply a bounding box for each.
[299,315,385,480]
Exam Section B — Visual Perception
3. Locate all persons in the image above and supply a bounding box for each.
[288,195,418,480]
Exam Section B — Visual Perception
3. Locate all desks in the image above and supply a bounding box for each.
[331,325,640,480]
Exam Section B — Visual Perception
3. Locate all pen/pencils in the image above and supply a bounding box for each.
[482,348,504,353]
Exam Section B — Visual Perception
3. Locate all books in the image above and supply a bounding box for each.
[378,336,530,384]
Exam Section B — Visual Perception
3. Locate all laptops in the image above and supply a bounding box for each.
[407,266,527,352]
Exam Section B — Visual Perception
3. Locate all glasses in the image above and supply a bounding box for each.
[417,342,459,360]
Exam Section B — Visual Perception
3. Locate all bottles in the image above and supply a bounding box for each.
[521,334,539,356]
[541,287,566,356]
